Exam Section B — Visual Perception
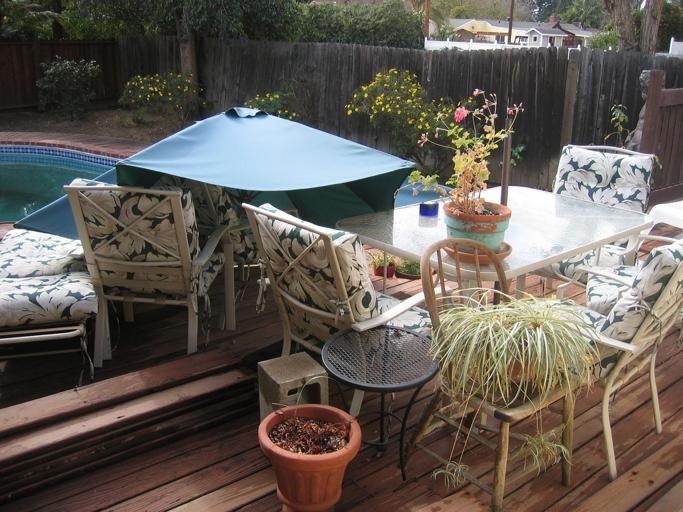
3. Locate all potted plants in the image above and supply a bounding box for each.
[259,377,362,510]
[365,249,396,277]
[425,287,602,489]
[396,258,433,279]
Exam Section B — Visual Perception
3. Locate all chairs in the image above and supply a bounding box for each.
[0,320,94,392]
[242,203,453,419]
[516,144,652,300]
[556,242,683,480]
[111,174,298,331]
[64,185,236,369]
[402,237,598,512]
[586,233,683,316]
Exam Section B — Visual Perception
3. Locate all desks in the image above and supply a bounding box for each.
[335,185,653,310]
[322,324,442,453]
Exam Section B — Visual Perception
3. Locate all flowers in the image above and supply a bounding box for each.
[417,89,524,216]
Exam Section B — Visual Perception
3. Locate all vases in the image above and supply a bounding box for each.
[444,200,510,254]
